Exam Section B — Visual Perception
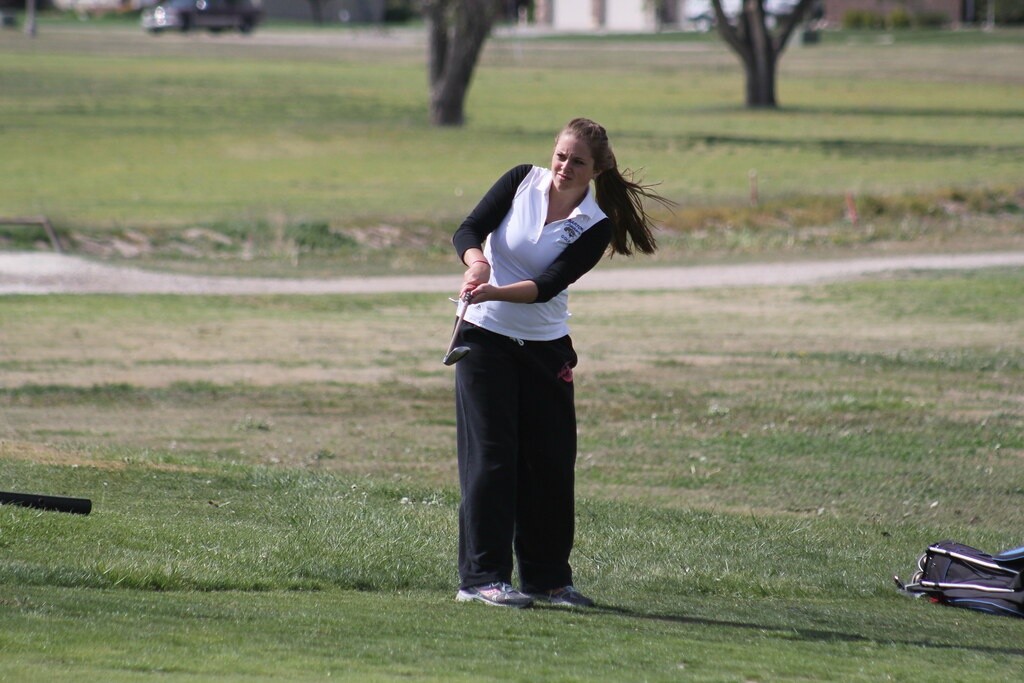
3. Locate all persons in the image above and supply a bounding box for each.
[451,117,678,612]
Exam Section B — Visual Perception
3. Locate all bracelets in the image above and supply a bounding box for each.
[473,260,491,267]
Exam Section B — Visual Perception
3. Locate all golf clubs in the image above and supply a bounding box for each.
[442,292,473,367]
[894,554,927,588]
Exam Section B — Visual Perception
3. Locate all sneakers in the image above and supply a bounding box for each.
[520,584,593,607]
[456,580,533,610]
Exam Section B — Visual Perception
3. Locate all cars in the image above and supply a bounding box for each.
[141,0,265,34]
[685,0,800,33]
